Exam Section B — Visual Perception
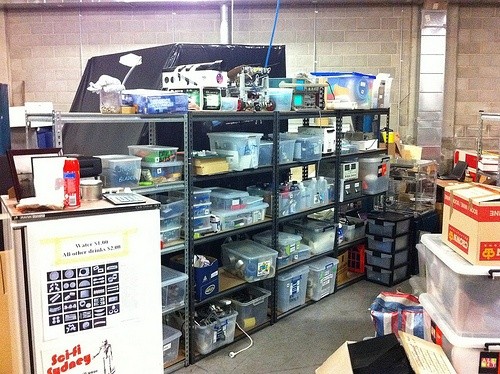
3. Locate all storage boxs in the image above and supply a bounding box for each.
[93,60,500,374]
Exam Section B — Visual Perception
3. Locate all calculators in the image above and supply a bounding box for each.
[102,193,145,205]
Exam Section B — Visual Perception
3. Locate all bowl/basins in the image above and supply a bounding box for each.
[80,180,103,201]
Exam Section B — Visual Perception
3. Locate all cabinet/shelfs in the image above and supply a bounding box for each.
[274,110,340,322]
[363,211,412,287]
[23,109,190,374]
[189,108,277,365]
[390,159,437,215]
[305,106,390,292]
[477,109,500,187]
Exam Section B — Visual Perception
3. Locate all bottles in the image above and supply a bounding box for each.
[63,158,81,209]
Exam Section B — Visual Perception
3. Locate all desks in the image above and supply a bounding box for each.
[0,193,166,374]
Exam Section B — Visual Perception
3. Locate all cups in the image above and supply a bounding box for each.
[99,87,121,114]
